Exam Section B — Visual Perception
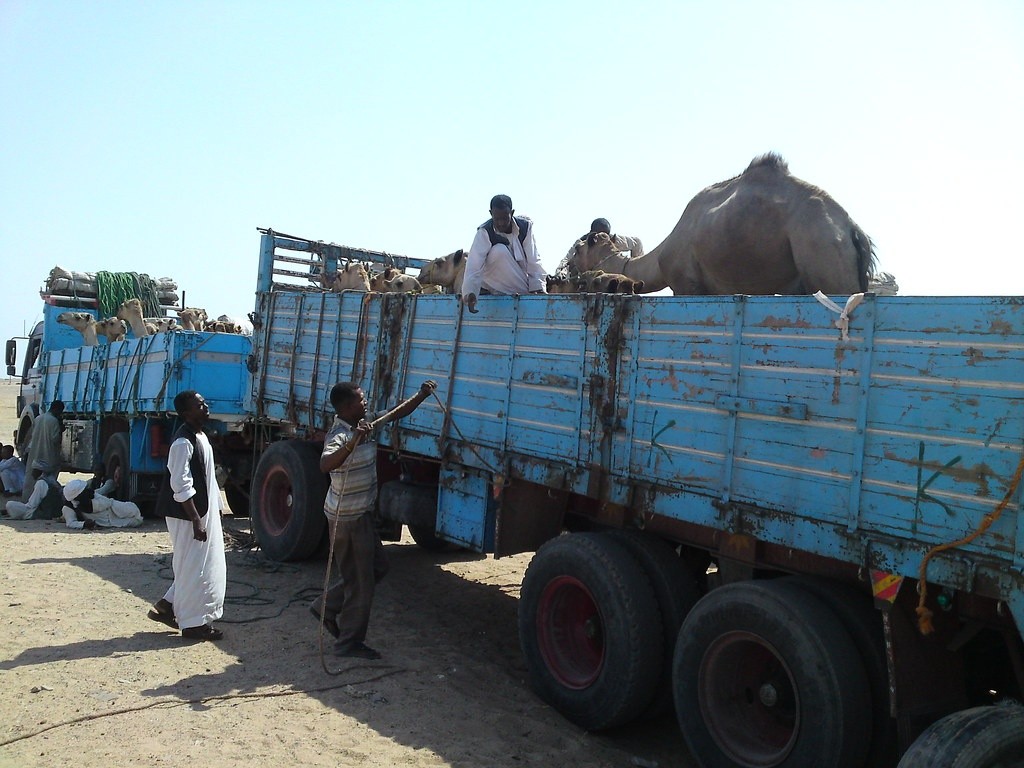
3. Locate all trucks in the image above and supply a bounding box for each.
[4,265,270,518]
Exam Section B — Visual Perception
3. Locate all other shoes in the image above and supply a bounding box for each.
[3,489,20,497]
[338,644,380,659]
[309,606,339,640]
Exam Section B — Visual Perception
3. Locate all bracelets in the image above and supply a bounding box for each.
[345,443,351,452]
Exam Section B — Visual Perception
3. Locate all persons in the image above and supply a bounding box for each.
[555,217,644,277]
[146,390,227,641]
[0,401,143,530]
[319,379,438,660]
[462,195,546,313]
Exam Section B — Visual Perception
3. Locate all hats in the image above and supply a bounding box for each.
[62,479,88,501]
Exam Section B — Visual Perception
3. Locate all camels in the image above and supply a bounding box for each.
[54,297,243,347]
[319,153,878,297]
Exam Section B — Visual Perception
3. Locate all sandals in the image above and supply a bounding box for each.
[147,600,179,628]
[182,626,223,639]
